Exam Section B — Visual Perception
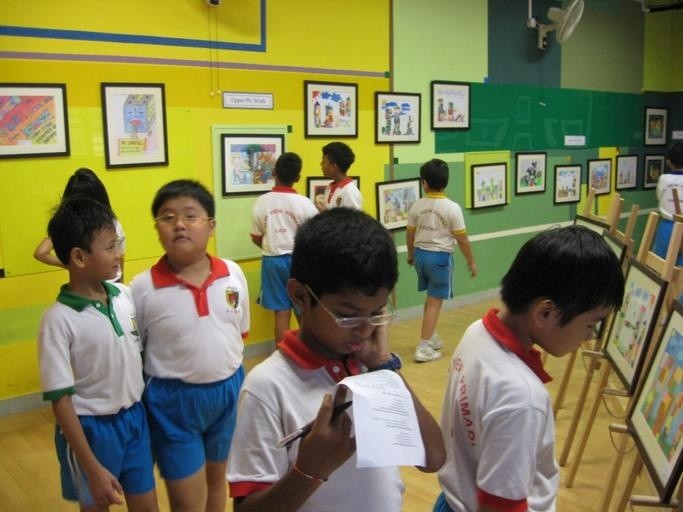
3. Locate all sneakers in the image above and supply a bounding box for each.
[414,346,441,361]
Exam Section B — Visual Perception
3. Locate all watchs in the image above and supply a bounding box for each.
[366,352,402,372]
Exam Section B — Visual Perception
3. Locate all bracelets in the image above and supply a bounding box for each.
[291,463,328,484]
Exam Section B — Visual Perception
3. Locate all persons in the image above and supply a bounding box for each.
[226,206,447,512]
[36,197,163,512]
[651,140,683,306]
[432,224,625,512]
[315,141,364,211]
[405,159,477,363]
[32,168,126,284]
[127,179,250,512]
[250,152,319,350]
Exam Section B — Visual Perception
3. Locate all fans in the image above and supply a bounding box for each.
[528,0,584,50]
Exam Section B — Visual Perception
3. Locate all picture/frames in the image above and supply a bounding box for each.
[303,80,359,138]
[100,81,169,167]
[0,82,71,157]
[572,212,683,503]
[220,132,285,195]
[430,80,471,131]
[376,178,421,231]
[470,106,671,210]
[306,176,360,200]
[373,89,421,143]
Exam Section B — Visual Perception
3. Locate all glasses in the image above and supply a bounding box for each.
[154,213,212,225]
[304,282,396,328]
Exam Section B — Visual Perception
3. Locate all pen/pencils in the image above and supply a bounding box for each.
[275,401,353,449]
